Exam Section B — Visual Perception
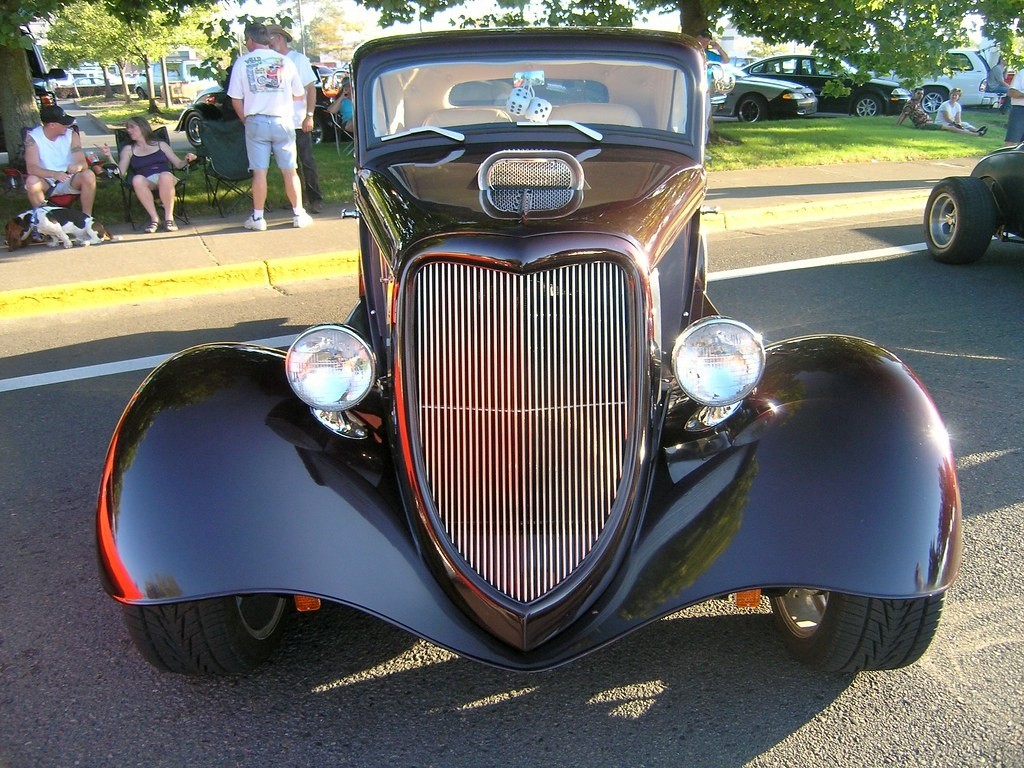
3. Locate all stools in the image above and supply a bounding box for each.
[982,93,1008,109]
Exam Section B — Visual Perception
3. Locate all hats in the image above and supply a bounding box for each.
[266,24,292,42]
[697,29,712,40]
[41,105,75,125]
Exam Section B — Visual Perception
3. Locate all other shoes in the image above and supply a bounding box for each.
[977,125,988,135]
[311,201,323,213]
[33,235,45,242]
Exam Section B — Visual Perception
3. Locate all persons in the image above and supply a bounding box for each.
[24,106,97,242]
[1004,68,1024,147]
[327,76,355,133]
[896,87,985,137]
[934,88,986,132]
[695,29,731,161]
[266,24,325,214]
[94,116,198,233]
[226,23,314,231]
[986,56,1012,114]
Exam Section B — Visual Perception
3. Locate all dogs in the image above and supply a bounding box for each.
[7,206,112,249]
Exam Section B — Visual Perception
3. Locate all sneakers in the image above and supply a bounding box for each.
[292,208,313,227]
[244,213,267,231]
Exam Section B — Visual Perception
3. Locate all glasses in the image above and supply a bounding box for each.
[915,92,924,95]
[242,37,249,45]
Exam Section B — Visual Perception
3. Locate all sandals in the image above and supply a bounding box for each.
[143,221,162,233]
[163,220,178,232]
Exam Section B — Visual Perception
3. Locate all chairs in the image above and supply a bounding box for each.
[549,103,643,128]
[100,127,192,232]
[801,68,808,74]
[3,126,105,208]
[421,107,513,128]
[193,120,270,219]
[322,76,357,157]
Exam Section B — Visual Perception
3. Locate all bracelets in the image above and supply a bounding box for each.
[306,112,314,117]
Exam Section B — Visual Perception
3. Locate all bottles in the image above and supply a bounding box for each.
[88,151,103,174]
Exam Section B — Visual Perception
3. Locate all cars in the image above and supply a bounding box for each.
[53,58,217,102]
[706,42,1018,125]
[90,23,965,684]
[312,58,355,108]
[18,23,67,119]
[922,138,1024,265]
[170,78,354,150]
